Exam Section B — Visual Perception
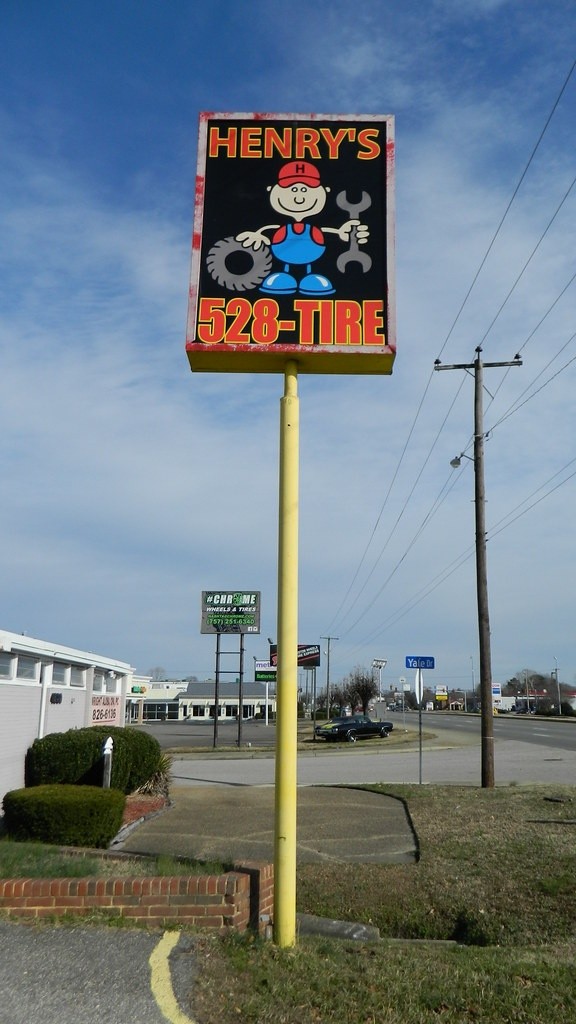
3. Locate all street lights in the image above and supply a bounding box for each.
[523,670,530,713]
[553,656,561,715]
[371,658,387,703]
[329,687,338,708]
[469,656,478,712]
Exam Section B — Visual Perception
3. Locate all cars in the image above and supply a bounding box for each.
[532,703,539,712]
[344,707,351,712]
[517,708,531,714]
[369,705,374,712]
[401,678,406,731]
[335,704,341,710]
[323,703,331,708]
[315,715,393,742]
[392,705,409,712]
[386,707,392,711]
[355,705,364,712]
